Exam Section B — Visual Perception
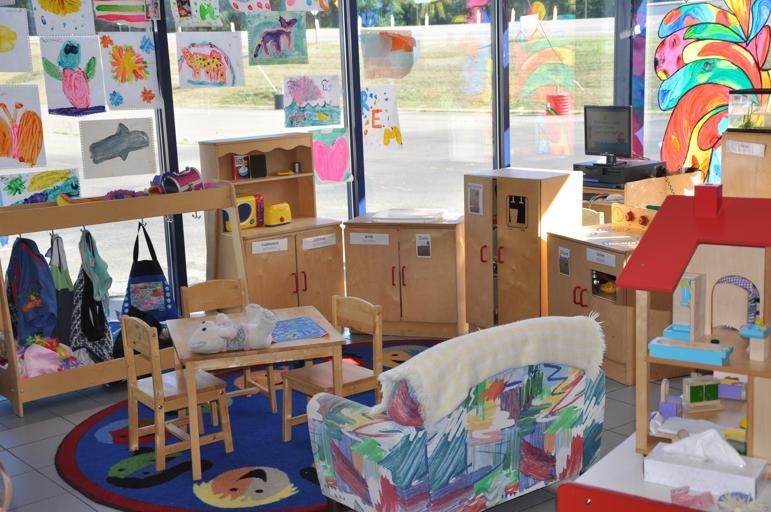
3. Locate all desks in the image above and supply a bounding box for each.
[166,304,347,481]
[556,428,771,512]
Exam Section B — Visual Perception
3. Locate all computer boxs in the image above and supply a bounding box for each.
[573,160,666,189]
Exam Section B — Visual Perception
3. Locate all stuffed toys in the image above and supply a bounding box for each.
[188,303,276,355]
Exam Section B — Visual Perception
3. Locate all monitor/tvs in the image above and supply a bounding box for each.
[584,105,633,166]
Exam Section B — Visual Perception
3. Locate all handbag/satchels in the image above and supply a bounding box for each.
[121,221,179,330]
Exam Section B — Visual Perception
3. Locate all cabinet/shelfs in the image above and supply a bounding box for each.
[721,127,771,199]
[0,178,251,418]
[343,209,469,340]
[463,166,578,334]
[546,202,691,387]
[582,171,701,223]
[198,131,345,334]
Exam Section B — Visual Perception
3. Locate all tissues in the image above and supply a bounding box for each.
[642,430,767,502]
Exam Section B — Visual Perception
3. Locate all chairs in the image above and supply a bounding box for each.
[307,309,608,511]
[180,278,278,427]
[120,313,234,473]
[279,294,384,443]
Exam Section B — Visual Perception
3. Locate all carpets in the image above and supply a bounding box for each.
[55,336,444,512]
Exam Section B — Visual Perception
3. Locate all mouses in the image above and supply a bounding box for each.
[607,194,624,200]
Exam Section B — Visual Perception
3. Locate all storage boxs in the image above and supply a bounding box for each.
[728,89,771,132]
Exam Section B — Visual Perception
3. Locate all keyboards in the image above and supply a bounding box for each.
[583,193,596,199]
